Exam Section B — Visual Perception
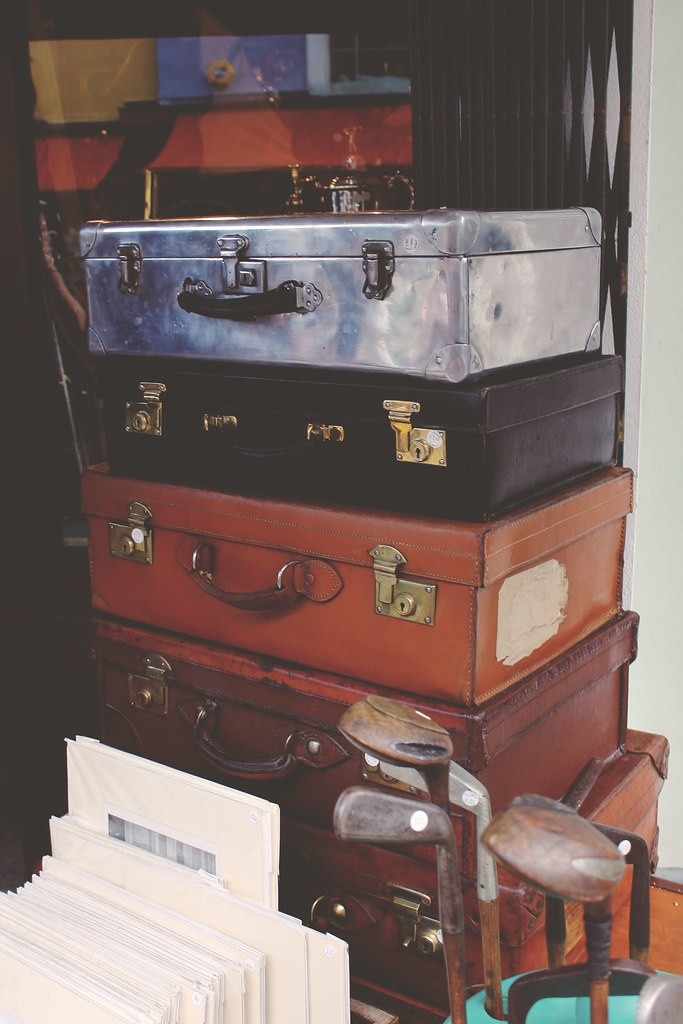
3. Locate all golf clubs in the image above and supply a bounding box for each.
[330,692,683,1024]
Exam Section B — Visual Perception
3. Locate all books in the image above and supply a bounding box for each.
[0,735,351,1023]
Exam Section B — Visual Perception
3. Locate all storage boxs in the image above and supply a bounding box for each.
[119,93,413,171]
[28,37,154,127]
[35,132,124,192]
[156,33,332,105]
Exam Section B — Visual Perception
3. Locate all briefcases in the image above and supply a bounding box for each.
[79,208,669,990]
